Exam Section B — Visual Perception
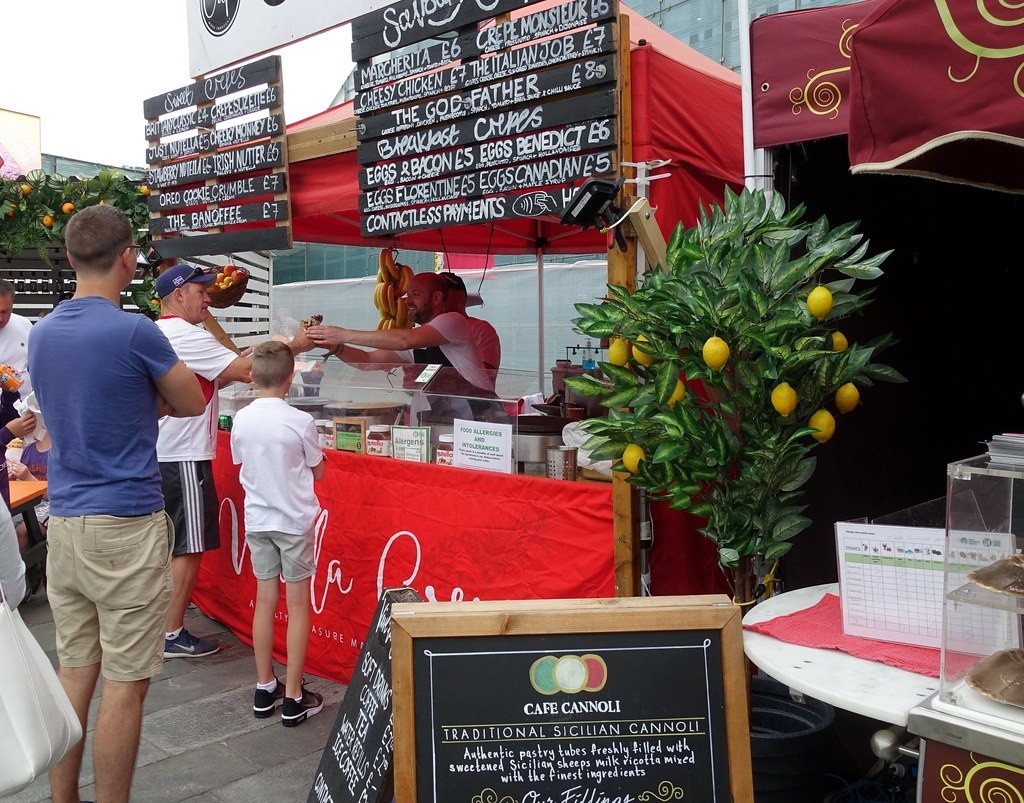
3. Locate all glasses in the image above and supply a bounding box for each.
[178,267,204,290]
[119,244,141,258]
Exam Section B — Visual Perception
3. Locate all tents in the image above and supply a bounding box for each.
[739,0,1023,197]
[151,0,744,595]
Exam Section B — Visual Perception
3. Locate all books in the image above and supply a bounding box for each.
[986,433,1024,468]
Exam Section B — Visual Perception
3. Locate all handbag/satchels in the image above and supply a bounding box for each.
[0,587,83,796]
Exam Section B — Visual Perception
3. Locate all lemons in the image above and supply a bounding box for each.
[607,285,860,476]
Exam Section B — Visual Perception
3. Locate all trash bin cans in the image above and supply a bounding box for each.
[747,673,838,803]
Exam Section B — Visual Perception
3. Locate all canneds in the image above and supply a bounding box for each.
[0,363,24,393]
[314,420,334,450]
[219,415,233,432]
[366,424,392,458]
[435,433,454,467]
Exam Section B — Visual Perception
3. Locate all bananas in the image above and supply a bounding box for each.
[372,248,416,331]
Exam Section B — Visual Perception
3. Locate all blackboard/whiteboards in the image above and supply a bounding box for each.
[381,591,758,803]
[306,584,426,803]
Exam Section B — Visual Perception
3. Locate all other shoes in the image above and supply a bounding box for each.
[22,570,34,603]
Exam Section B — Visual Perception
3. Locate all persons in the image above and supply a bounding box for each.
[230,341,328,727]
[28,205,208,803]
[0,279,49,555]
[305,273,500,409]
[153,265,318,659]
[438,272,501,392]
[0,493,26,610]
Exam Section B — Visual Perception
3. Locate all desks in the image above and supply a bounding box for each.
[8,480,47,590]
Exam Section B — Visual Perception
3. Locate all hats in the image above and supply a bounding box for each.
[439,272,466,291]
[155,264,216,299]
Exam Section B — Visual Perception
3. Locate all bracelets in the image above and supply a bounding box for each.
[332,343,344,357]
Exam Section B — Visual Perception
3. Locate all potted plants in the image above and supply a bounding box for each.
[562,184,909,802]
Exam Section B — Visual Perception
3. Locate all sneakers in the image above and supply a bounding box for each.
[281,678,323,727]
[253,667,286,718]
[162,627,221,658]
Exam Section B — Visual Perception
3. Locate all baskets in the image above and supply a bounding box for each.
[193,252,250,309]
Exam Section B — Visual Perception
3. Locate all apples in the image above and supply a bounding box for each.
[5,184,150,227]
[205,265,246,292]
